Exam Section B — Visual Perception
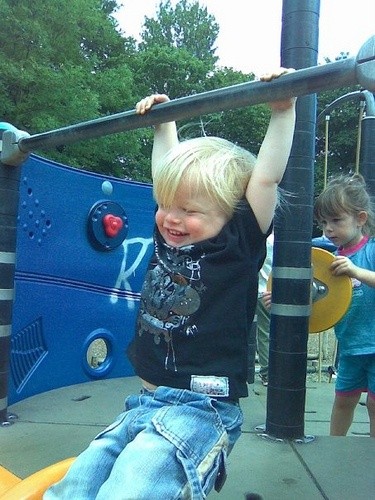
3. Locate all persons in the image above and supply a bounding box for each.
[256,228,274,385]
[262,174,375,437]
[42,67,299,500]
